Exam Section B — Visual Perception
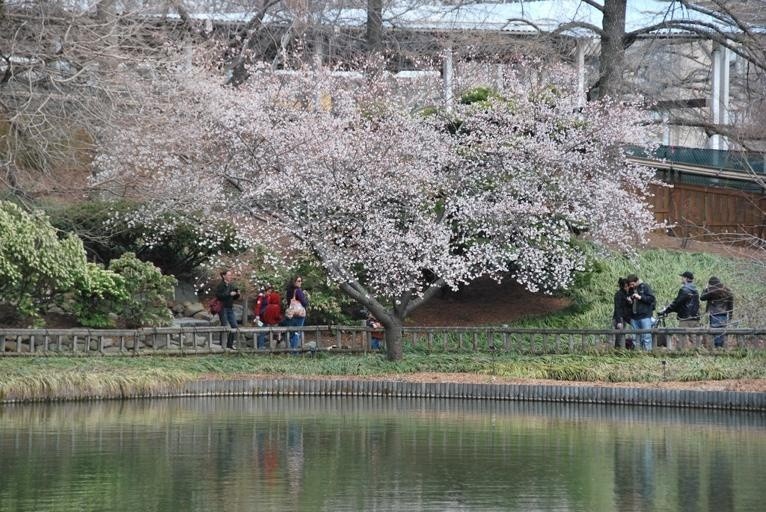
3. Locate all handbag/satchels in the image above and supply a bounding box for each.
[209,297,222,315]
[285,288,307,319]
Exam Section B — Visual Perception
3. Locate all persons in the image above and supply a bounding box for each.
[612,278,633,350]
[214,269,241,351]
[659,271,702,351]
[699,276,735,348]
[263,291,283,344]
[626,273,658,351]
[365,310,385,352]
[286,274,310,353]
[253,285,273,350]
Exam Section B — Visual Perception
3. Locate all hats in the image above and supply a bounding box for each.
[677,271,695,279]
